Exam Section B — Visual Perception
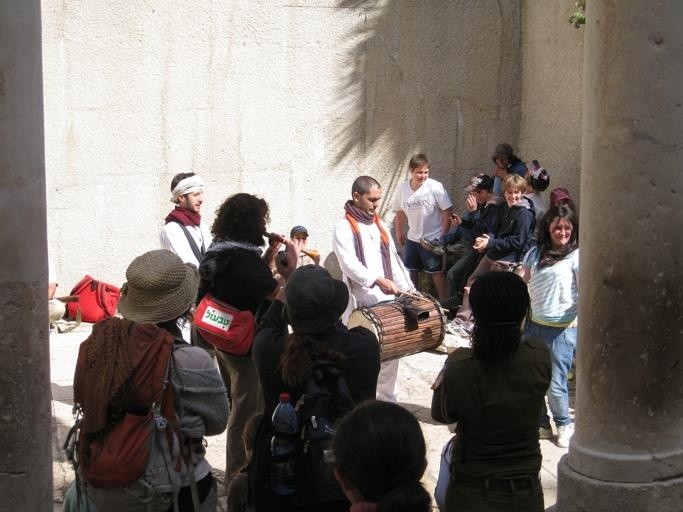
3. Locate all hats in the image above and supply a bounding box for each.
[551,189,572,202]
[462,174,492,191]
[527,159,549,191]
[285,265,349,335]
[291,226,308,237]
[117,249,200,326]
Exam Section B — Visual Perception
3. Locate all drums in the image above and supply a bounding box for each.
[347,293,446,362]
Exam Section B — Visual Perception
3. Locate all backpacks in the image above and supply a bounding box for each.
[67,276,120,323]
[61,401,181,512]
[250,341,368,512]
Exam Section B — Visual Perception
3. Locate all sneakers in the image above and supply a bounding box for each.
[528,422,572,448]
[445,321,474,338]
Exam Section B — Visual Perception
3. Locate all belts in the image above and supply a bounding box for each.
[453,474,539,489]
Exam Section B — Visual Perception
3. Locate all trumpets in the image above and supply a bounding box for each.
[263,231,320,265]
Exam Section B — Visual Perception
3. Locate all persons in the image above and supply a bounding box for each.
[159,172,215,345]
[56,246,233,512]
[227,264,432,512]
[392,143,582,448]
[198,193,304,485]
[333,176,416,308]
[289,226,320,265]
[430,270,553,511]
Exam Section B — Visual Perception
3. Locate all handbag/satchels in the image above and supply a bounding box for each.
[194,294,255,356]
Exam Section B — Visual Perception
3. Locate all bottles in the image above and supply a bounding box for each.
[267,395,295,461]
